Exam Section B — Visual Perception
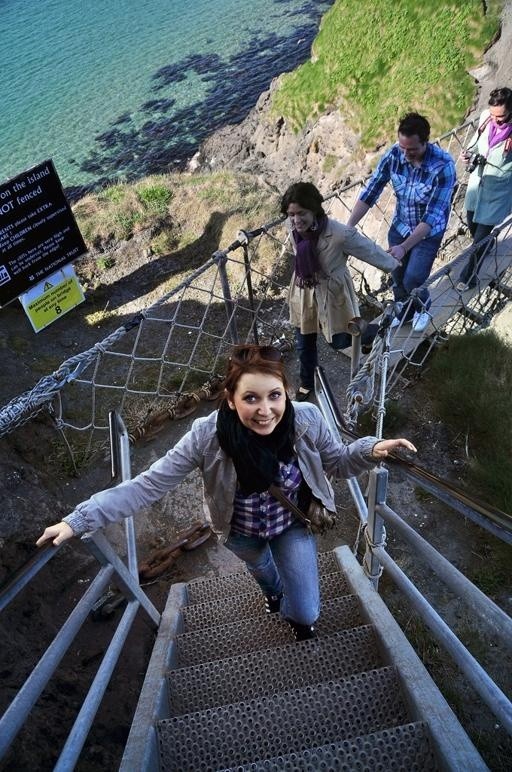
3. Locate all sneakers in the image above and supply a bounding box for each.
[285,617,315,641]
[390,310,429,331]
[362,345,372,354]
[456,282,469,291]
[264,594,283,613]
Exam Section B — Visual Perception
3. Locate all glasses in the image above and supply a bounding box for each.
[235,345,280,361]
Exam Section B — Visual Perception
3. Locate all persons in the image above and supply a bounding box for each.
[280,181,402,401]
[345,114,457,333]
[36,347,417,643]
[457,87,512,291]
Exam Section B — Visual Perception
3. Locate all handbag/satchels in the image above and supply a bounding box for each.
[304,497,338,536]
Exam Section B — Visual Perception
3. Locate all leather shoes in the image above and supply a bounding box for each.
[296,389,312,401]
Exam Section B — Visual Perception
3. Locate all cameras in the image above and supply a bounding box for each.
[464,150,487,173]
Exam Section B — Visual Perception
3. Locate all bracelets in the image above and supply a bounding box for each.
[400,245,407,255]
